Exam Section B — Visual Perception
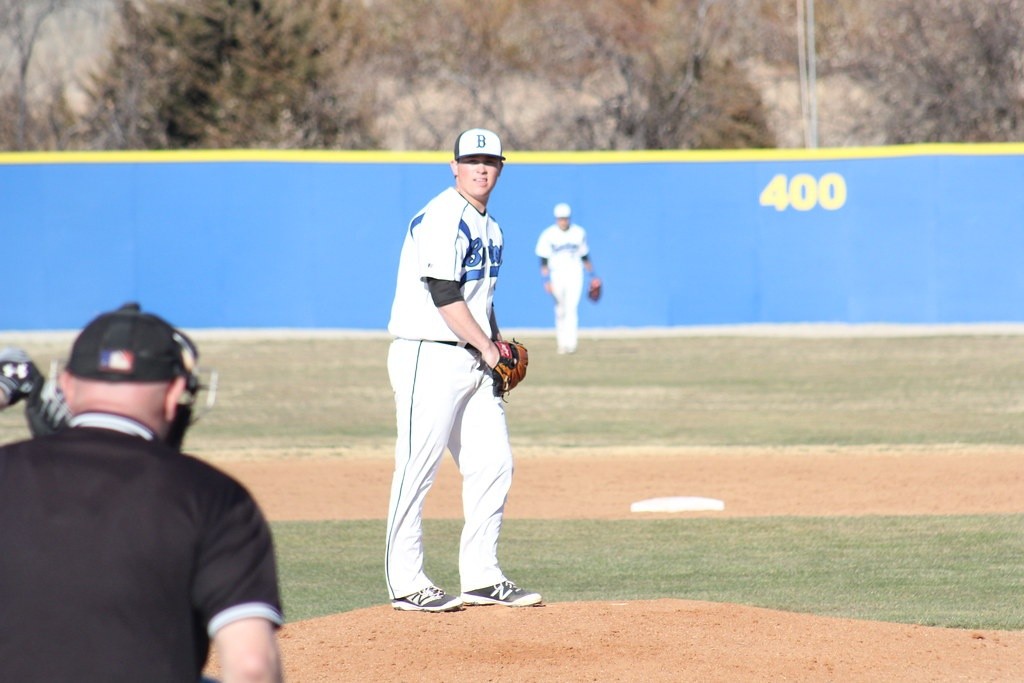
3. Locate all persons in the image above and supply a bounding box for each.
[0,300,285,683]
[536,203,602,353]
[384,128,542,611]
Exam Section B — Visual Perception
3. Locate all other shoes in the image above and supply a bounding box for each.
[567,345,576,353]
[557,346,566,354]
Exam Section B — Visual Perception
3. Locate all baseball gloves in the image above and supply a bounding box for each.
[492,340,528,397]
[589,278,602,301]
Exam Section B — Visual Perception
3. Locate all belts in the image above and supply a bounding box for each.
[435,341,474,350]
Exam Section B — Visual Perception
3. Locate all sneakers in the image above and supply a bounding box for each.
[462,580,542,606]
[392,586,463,612]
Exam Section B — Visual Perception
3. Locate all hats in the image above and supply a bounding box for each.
[454,127,506,162]
[66,307,195,387]
[553,202,570,218]
